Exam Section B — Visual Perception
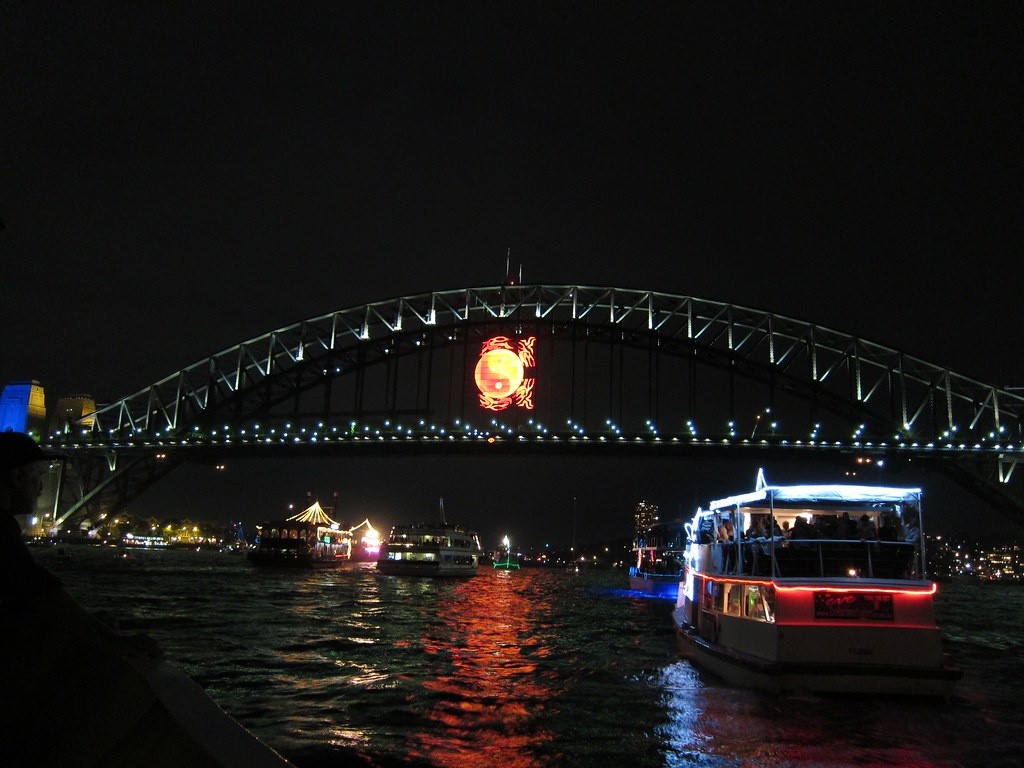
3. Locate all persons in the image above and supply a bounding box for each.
[718,510,922,578]
[0,432,164,768]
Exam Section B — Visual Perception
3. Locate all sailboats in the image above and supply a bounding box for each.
[492,536,520,571]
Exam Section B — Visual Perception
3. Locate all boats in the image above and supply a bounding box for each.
[671,468,963,702]
[626,539,684,596]
[247,498,354,570]
[122,531,174,551]
[377,519,486,578]
